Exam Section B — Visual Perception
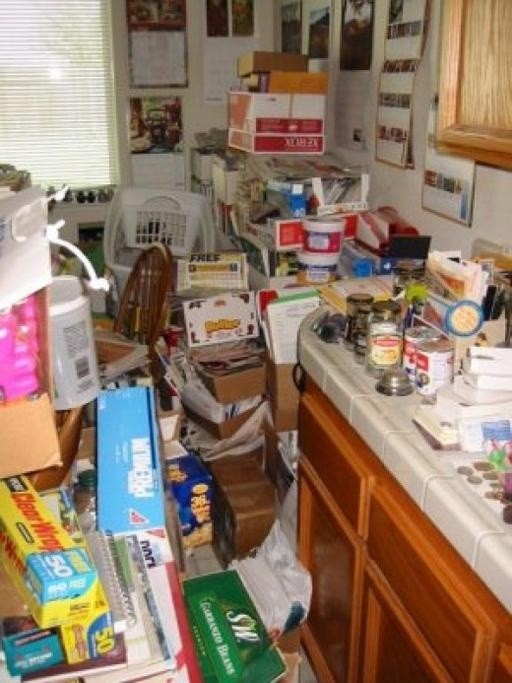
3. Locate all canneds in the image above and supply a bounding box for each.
[347,294,373,349]
[415,342,453,400]
[367,300,404,379]
[355,307,370,355]
[404,327,442,384]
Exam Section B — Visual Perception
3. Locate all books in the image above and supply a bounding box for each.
[411,346,512,451]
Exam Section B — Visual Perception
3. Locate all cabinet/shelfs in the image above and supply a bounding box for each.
[290,298,512,682]
[430,0,512,170]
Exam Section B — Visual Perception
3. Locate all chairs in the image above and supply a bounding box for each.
[114,241,171,384]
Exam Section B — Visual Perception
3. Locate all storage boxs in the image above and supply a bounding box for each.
[221,86,326,158]
[1,285,67,480]
[28,404,86,494]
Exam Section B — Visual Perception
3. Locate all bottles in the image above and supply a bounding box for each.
[343,290,404,377]
